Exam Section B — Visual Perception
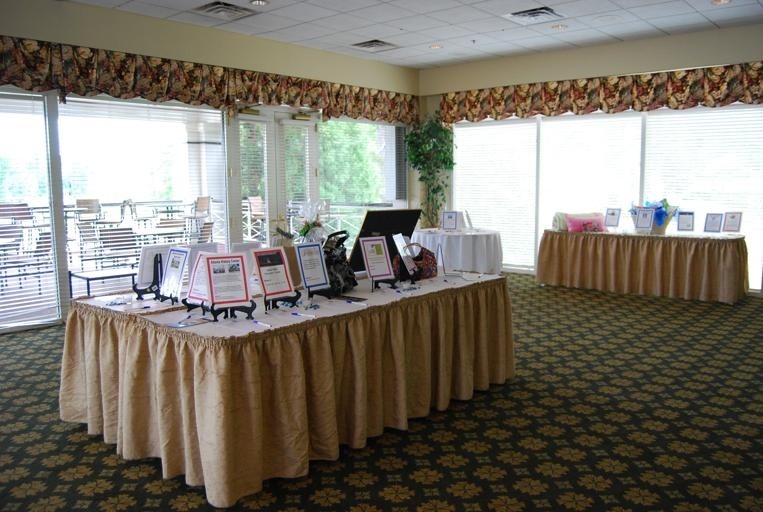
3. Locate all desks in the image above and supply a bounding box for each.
[535,230,749,305]
[120,269,517,508]
[409,227,505,277]
[58,268,367,448]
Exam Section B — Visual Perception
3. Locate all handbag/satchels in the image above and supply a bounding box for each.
[393,242,437,279]
[320,230,357,298]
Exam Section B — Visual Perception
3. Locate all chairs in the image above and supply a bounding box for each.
[0,197,333,296]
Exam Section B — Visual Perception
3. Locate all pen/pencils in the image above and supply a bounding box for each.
[255,321,271,327]
[396,290,404,295]
[292,313,314,317]
[444,280,456,284]
[347,301,368,306]
[178,316,190,324]
[135,306,150,309]
